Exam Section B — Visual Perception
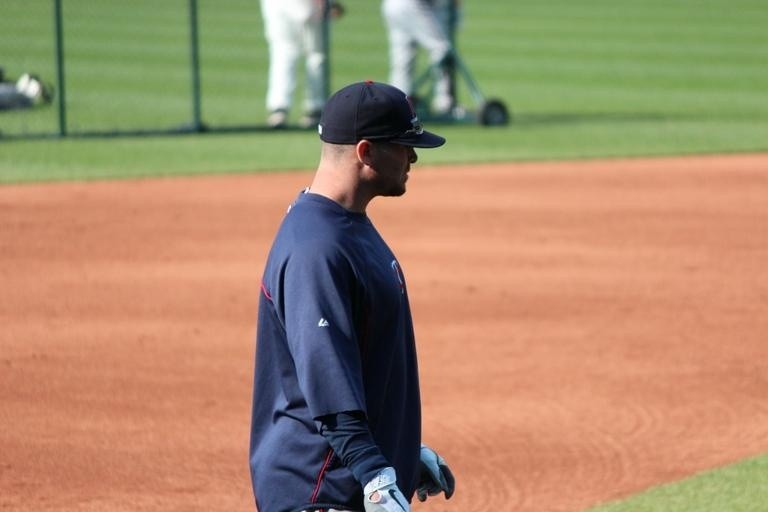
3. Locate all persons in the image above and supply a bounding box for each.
[259,1,339,131]
[249,79,457,511]
[382,0,468,125]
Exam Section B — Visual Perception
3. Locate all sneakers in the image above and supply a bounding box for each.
[265,108,318,133]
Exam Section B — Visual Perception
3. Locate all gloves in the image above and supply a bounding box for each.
[412,443,456,504]
[359,466,410,512]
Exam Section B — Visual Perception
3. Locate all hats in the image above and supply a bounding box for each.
[319,79,452,154]
